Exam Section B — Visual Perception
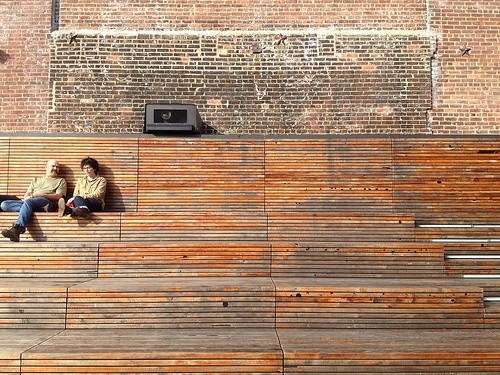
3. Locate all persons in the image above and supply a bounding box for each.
[58,158,106,220]
[1,158,67,242]
